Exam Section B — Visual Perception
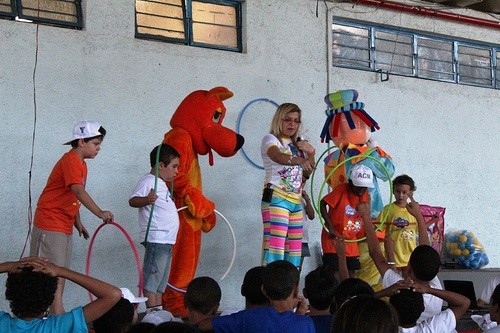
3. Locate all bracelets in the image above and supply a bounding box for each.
[287,153,297,165]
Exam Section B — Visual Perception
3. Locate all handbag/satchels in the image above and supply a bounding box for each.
[418,204,446,257]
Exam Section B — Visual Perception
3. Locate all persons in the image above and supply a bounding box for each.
[129,143,181,314]
[357,192,444,325]
[375,174,430,278]
[260,102,317,275]
[30,120,114,314]
[0,256,500,333]
[320,165,374,287]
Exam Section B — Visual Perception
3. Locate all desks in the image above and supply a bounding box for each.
[436,268,500,306]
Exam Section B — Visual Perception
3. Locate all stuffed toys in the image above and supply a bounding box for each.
[161,87,244,318]
[320,89,395,303]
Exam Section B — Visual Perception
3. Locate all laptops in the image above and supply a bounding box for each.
[444,279,490,312]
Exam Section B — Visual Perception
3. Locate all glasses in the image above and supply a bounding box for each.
[282,118,301,123]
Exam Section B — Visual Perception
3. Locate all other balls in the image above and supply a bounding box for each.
[445,229,489,269]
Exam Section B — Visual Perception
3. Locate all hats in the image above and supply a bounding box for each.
[120,288,148,303]
[348,165,374,188]
[141,310,184,326]
[62,120,102,145]
[470,313,498,331]
